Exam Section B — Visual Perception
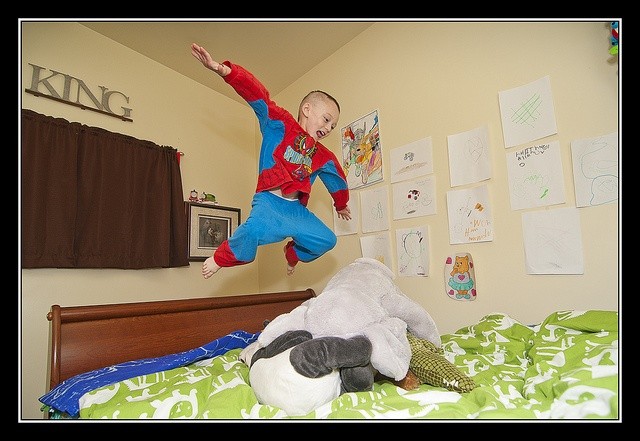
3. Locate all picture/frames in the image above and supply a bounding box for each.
[184,200,242,262]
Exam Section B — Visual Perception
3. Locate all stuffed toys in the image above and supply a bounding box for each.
[239,258,442,416]
[407,329,476,393]
[373,367,421,390]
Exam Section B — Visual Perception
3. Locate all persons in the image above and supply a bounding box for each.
[190,42,352,279]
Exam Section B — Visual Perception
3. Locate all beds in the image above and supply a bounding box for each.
[38,288,619,420]
